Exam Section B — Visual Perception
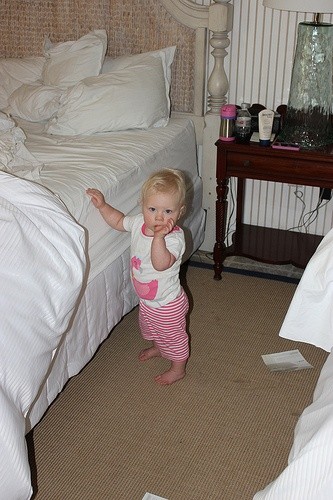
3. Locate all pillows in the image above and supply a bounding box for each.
[3,30,110,123]
[43,51,170,137]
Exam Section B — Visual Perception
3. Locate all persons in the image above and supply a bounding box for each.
[86,169,189,385]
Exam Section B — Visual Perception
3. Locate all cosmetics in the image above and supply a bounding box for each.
[258,109,274,145]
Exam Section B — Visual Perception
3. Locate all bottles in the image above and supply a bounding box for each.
[236,103,252,143]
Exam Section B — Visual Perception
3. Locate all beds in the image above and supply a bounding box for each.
[0,29,206,500]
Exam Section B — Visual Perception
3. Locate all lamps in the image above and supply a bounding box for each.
[263,0,333,151]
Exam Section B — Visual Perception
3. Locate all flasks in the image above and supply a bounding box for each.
[219,104,236,142]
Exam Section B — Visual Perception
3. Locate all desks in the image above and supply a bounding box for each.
[213,138,333,280]
